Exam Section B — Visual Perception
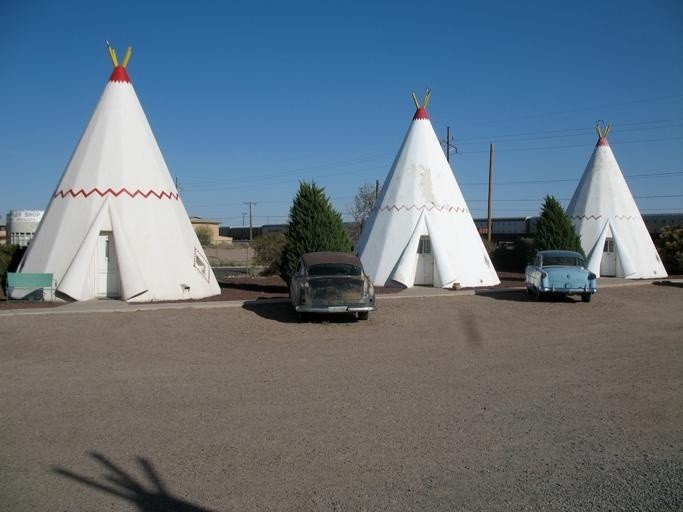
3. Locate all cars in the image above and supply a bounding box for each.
[521,248,599,303]
[287,251,377,323]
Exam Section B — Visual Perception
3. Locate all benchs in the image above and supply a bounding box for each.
[6,271,57,304]
